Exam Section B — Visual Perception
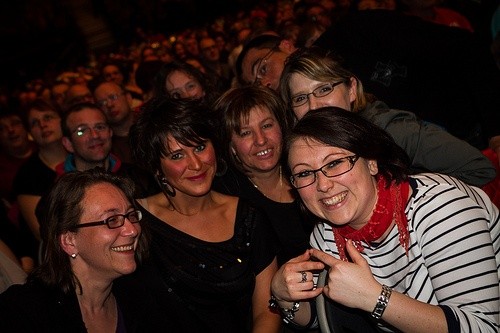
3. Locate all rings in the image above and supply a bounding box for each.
[300,271,306,283]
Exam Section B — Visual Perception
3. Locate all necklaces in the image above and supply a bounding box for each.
[247,165,283,202]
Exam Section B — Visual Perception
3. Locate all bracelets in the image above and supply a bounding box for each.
[371,284,391,319]
[268,295,300,314]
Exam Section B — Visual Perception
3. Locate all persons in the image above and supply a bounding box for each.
[0,0,500,333]
[271,106,500,333]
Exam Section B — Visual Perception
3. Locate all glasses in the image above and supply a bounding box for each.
[290,154,360,188]
[97,92,125,105]
[251,51,275,85]
[290,82,343,107]
[72,209,143,229]
[71,124,111,135]
